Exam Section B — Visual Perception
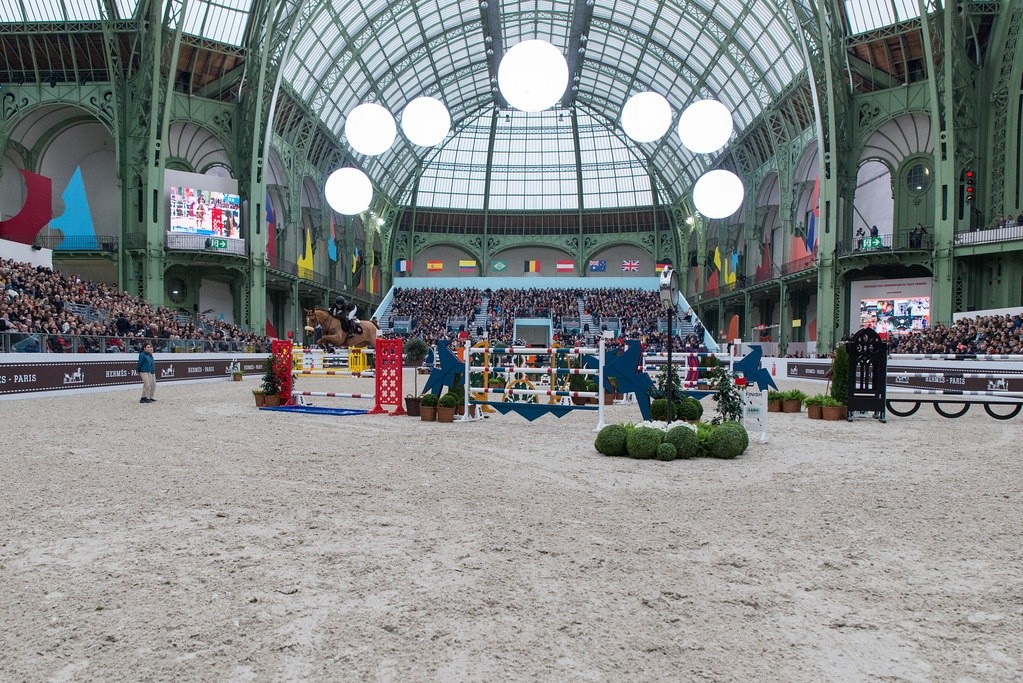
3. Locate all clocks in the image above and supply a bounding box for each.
[659,265,680,313]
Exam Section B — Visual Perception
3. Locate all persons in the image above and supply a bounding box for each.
[841,332,855,341]
[855,227,866,251]
[0,257,274,354]
[195,195,207,214]
[210,197,239,234]
[861,301,929,337]
[136,342,157,403]
[582,287,710,353]
[885,312,1023,355]
[912,222,928,248]
[485,287,591,347]
[995,211,1023,228]
[377,287,483,349]
[329,297,358,338]
[762,348,836,359]
[870,225,879,237]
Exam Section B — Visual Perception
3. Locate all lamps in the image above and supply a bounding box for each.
[481,0,510,123]
[325,167,373,215]
[693,169,744,218]
[621,91,672,142]
[402,96,451,147]
[678,99,732,154]
[559,0,595,122]
[498,39,568,113]
[345,103,396,156]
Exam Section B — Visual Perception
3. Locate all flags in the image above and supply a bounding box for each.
[556,259,574,272]
[622,259,639,272]
[523,261,540,272]
[589,259,607,272]
[395,260,412,272]
[492,261,508,271]
[426,259,443,272]
[655,260,673,275]
[459,260,476,272]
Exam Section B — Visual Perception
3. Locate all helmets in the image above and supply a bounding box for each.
[336,296,345,306]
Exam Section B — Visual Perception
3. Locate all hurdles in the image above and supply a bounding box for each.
[270,337,407,417]
[615,337,735,398]
[450,339,612,433]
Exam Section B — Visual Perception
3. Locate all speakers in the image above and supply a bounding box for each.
[33,244,42,250]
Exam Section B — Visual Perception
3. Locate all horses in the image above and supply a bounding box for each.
[304,305,380,354]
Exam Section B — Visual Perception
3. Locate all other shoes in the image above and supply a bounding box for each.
[150,398,157,401]
[140,396,151,403]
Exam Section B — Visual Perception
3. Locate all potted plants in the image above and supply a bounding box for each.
[488,377,506,388]
[232,370,245,380]
[768,390,847,421]
[252,356,280,408]
[570,357,622,406]
[405,337,475,423]
